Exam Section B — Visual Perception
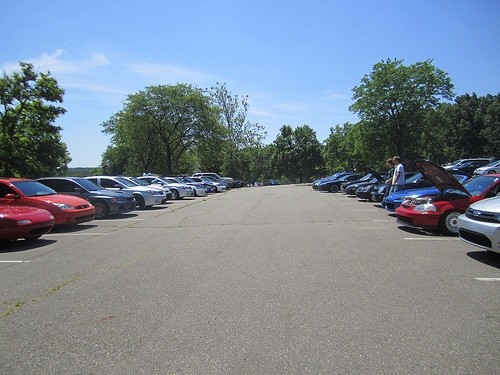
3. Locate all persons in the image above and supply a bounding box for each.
[374,158,395,208]
[389,155,405,212]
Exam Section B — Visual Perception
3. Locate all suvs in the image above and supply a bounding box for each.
[441,158,493,177]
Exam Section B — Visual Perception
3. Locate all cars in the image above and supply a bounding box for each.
[312,168,470,213]
[473,159,500,174]
[34,176,137,220]
[0,177,96,233]
[395,158,500,237]
[456,192,500,256]
[0,203,55,248]
[83,176,167,210]
[267,180,278,185]
[137,172,244,201]
